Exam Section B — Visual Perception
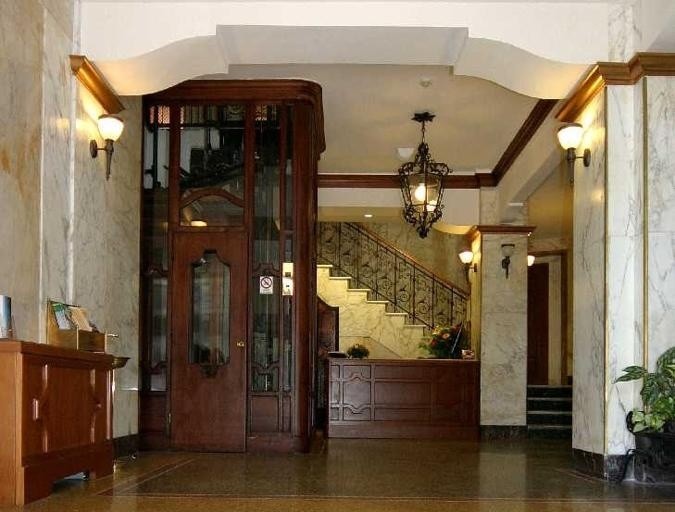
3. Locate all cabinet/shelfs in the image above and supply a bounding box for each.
[1,340,130,506]
[140,78,326,453]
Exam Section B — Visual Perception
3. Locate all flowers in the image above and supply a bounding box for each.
[418,321,471,359]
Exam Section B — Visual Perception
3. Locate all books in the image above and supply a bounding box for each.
[52,302,92,332]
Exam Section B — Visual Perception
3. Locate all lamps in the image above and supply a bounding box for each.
[400,111,452,239]
[501,243,515,278]
[458,251,477,272]
[555,124,593,167]
[89,115,125,181]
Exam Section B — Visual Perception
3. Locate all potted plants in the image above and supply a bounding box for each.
[613,347,673,487]
